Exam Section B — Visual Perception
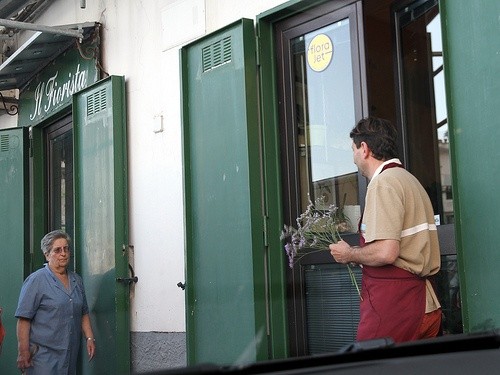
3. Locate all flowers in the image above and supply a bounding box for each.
[280,193,363,302]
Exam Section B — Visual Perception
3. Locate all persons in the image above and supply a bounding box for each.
[329,118,442,341]
[14,230,95,375]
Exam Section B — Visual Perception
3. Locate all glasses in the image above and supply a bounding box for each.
[53,246,70,253]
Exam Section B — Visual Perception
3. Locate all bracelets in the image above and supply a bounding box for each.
[86,337,95,342]
